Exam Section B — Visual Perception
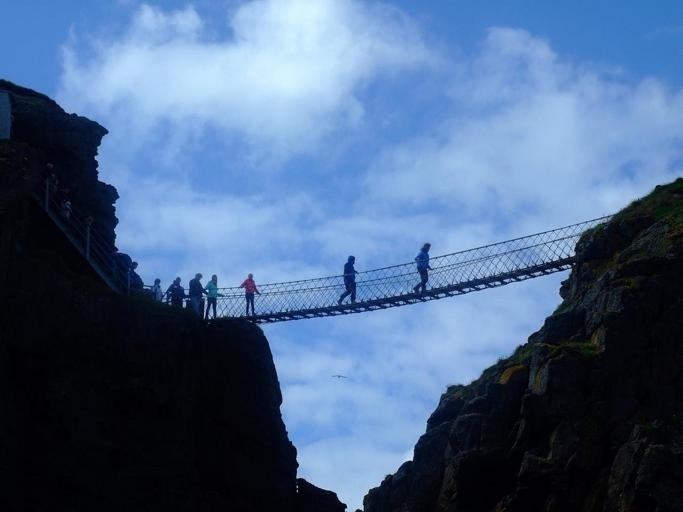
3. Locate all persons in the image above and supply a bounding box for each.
[203,274,224,319]
[412,242,434,294]
[149,277,164,301]
[168,277,186,306]
[241,273,262,317]
[337,256,359,305]
[38,162,142,292]
[189,273,208,316]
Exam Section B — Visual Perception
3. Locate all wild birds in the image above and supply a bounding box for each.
[331,375,349,378]
